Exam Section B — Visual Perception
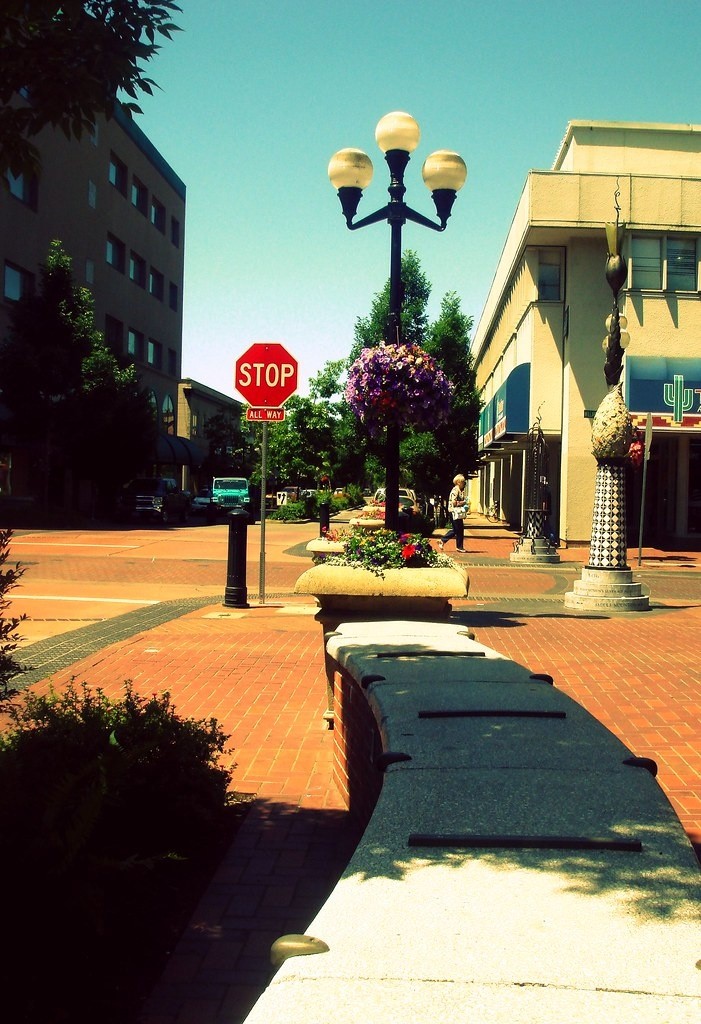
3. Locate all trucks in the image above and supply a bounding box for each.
[211,476,251,510]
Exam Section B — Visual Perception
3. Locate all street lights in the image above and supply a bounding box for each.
[326,110,469,537]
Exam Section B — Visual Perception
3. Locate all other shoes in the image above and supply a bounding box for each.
[456,548,466,552]
[436,540,443,550]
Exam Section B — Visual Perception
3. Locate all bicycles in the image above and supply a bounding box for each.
[485,501,500,523]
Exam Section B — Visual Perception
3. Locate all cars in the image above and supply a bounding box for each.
[282,486,323,502]
[334,488,344,499]
[373,487,421,519]
[191,488,210,511]
[124,479,192,526]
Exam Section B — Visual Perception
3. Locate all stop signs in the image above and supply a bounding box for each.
[235,342,298,411]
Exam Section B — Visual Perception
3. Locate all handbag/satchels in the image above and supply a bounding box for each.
[452,503,466,520]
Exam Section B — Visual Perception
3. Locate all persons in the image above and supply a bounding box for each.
[436,474,467,553]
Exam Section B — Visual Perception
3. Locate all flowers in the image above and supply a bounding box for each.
[368,499,403,508]
[315,520,375,544]
[396,507,436,525]
[310,527,457,607]
[343,340,457,439]
[352,509,385,520]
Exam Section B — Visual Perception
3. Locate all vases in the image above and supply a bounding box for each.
[398,520,437,537]
[307,537,351,557]
[363,506,403,512]
[348,519,385,535]
[291,562,471,723]
